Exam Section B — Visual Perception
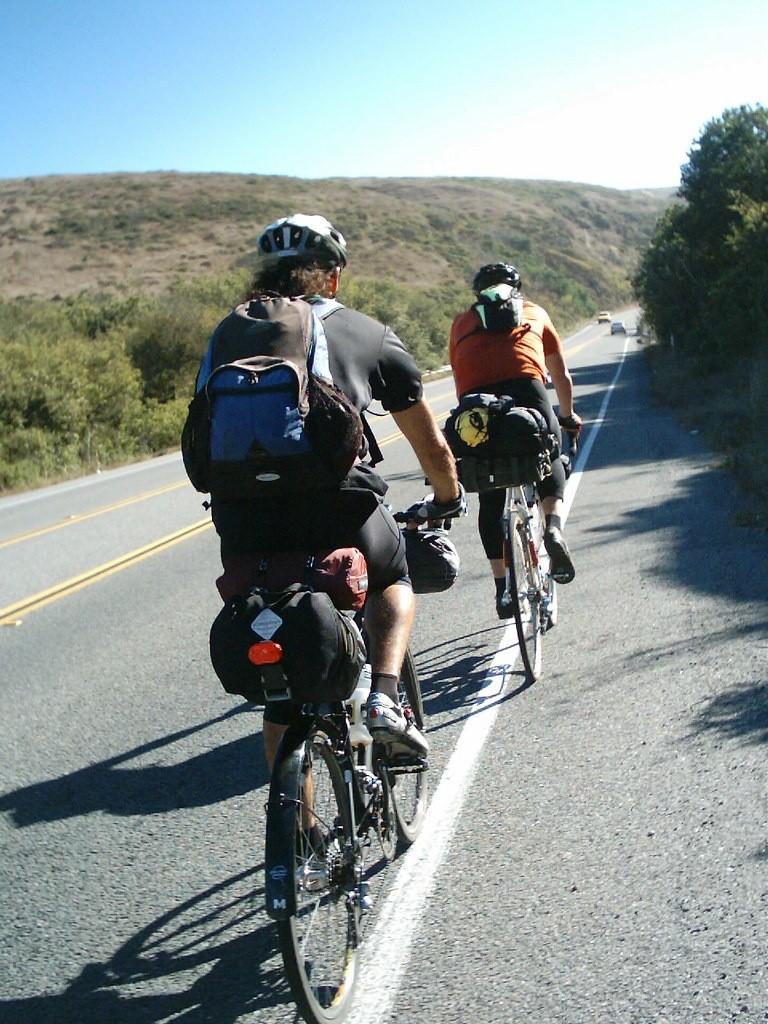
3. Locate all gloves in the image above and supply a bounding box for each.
[558,410,581,432]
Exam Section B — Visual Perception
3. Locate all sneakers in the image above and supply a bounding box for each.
[295,860,327,892]
[365,691,429,759]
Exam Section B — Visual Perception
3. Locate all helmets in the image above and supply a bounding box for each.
[257,214,347,272]
[472,263,521,299]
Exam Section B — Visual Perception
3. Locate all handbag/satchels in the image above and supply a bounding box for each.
[209,583,368,704]
[456,448,552,494]
[443,392,547,457]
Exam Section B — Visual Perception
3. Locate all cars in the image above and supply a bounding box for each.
[598,310,611,323]
[610,321,627,335]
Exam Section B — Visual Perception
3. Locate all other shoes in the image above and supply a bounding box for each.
[497,591,514,619]
[543,525,575,584]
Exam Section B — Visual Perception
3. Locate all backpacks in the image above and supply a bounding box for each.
[180,290,364,499]
[471,283,523,331]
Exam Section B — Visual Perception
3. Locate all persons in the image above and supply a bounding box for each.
[448,261,583,620]
[181,212,468,883]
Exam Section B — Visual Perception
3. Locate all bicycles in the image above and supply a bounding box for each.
[486,425,578,685]
[237,511,431,1024]
